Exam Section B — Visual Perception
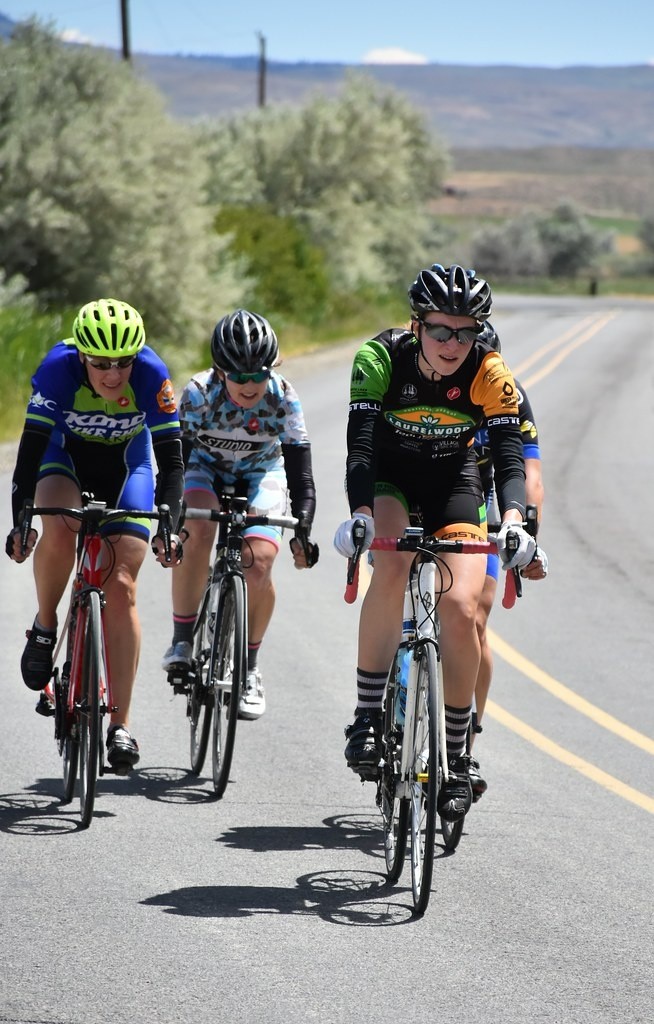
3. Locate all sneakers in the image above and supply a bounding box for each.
[344,716,383,766]
[465,756,488,793]
[436,756,472,823]
[106,726,140,765]
[162,641,193,671]
[237,666,266,719]
[21,611,58,691]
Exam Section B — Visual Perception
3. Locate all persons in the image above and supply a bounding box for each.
[334,264,548,824]
[160,308,319,719]
[6,298,184,768]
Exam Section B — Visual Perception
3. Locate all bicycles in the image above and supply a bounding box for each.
[167,487,313,796]
[343,505,540,914]
[19,490,173,823]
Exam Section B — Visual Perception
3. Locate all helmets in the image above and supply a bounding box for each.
[477,320,501,353]
[72,298,146,358]
[210,309,279,373]
[407,263,492,322]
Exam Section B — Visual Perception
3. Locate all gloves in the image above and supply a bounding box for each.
[334,513,375,558]
[496,522,536,571]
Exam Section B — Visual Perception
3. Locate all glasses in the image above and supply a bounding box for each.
[223,371,270,384]
[418,319,485,344]
[83,354,136,370]
